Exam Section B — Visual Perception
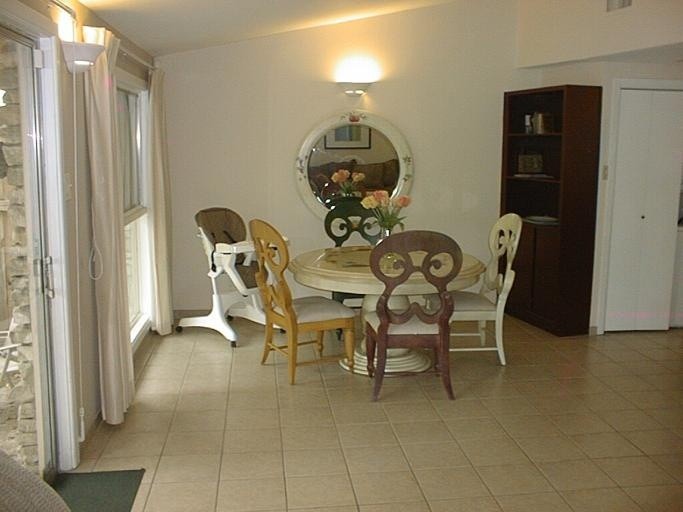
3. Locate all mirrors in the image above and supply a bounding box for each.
[295,108,414,230]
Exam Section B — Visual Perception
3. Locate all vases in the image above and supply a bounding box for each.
[382,227,394,262]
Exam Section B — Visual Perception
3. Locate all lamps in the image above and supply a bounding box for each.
[335,82,377,98]
[62,41,106,76]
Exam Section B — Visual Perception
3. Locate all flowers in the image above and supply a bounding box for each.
[360,189,411,231]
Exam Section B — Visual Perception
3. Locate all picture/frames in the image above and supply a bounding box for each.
[324,128,371,149]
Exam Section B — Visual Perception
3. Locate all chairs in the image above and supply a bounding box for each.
[176,206,286,348]
[323,201,392,341]
[423,212,523,366]
[249,218,356,385]
[363,230,463,401]
[0,447,72,512]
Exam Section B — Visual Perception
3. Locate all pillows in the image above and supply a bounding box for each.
[308,159,399,197]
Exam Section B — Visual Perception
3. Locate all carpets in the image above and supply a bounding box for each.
[52,469,146,512]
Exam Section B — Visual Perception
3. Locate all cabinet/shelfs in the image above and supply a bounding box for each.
[491,85,603,338]
